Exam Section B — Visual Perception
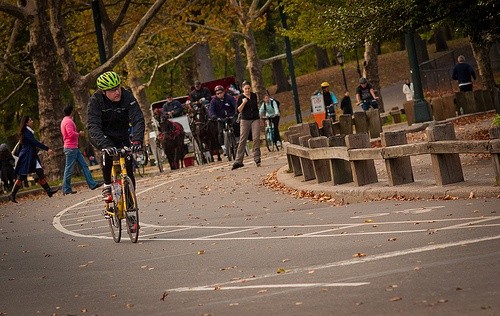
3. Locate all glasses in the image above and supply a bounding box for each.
[217,92,223,95]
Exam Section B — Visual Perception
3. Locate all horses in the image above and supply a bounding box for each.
[188,102,222,165]
[155,113,185,172]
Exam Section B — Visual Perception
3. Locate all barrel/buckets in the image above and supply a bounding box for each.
[184,156,194,167]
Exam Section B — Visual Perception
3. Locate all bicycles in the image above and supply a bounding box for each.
[101,146,144,243]
[358,99,378,110]
[207,115,236,162]
[260,115,283,152]
[325,103,336,124]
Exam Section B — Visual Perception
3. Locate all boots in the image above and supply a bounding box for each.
[40,182,59,198]
[6,183,20,204]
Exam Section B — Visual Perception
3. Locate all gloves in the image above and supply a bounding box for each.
[129,144,142,153]
[101,146,119,157]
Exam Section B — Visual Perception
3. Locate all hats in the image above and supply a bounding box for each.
[166,93,172,98]
[194,80,201,85]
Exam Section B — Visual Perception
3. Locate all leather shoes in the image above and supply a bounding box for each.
[90,182,105,190]
[62,190,78,196]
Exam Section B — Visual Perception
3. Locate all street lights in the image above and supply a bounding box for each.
[336,51,348,91]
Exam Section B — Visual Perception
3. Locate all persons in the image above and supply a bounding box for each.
[159,80,281,170]
[60,105,104,196]
[402,78,414,101]
[0,115,59,203]
[340,90,354,115]
[86,71,145,234]
[355,78,379,112]
[317,82,340,123]
[451,55,476,91]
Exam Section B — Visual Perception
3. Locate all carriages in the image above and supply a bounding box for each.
[186,75,249,164]
[151,95,202,173]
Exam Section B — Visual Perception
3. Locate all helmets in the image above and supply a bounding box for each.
[320,82,330,88]
[214,85,224,91]
[359,77,367,84]
[96,71,122,91]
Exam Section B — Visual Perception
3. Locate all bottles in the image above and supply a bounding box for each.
[114,183,120,198]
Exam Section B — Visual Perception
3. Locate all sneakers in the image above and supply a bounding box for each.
[276,140,282,147]
[101,186,114,202]
[127,216,141,234]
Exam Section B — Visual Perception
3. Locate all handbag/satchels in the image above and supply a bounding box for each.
[11,139,22,161]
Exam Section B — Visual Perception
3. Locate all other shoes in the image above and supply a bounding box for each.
[231,161,245,171]
[255,161,261,167]
